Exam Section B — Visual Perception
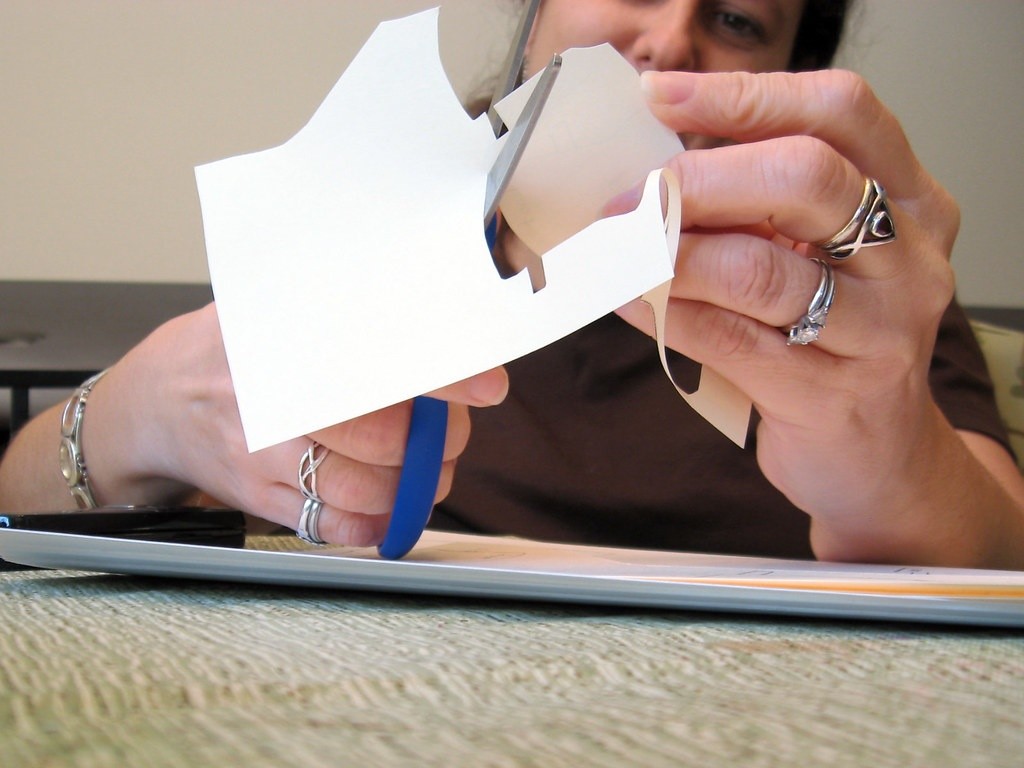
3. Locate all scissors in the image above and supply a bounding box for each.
[374,0,563,561]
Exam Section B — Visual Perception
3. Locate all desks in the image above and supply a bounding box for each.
[0,282,214,443]
[0,530,1024,768]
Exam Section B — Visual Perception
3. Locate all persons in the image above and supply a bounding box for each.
[0,0,1024,570]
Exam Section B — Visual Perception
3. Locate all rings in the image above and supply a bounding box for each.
[785,257,836,348]
[296,499,330,548]
[297,440,330,504]
[821,178,894,259]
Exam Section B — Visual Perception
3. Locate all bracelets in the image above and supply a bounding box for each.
[58,372,103,508]
